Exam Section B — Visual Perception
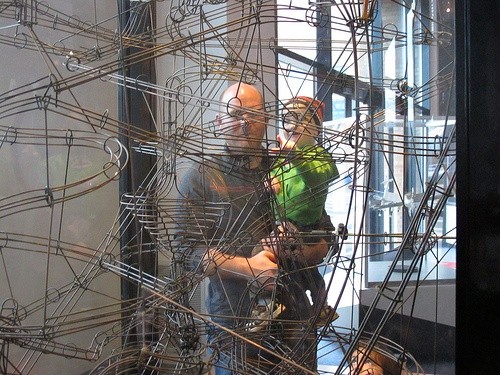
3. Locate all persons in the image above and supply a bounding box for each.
[240,95,340,333]
[171,81,336,375]
[347,340,402,375]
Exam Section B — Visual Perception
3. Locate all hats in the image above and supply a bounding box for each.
[287,96,325,137]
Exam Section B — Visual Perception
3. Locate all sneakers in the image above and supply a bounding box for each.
[310,304,339,329]
[243,299,285,334]
